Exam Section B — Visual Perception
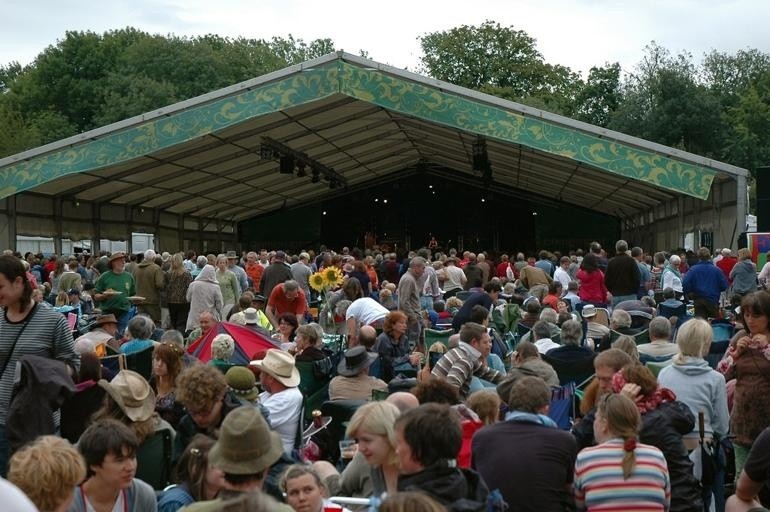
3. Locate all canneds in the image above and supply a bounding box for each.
[312,410,323,428]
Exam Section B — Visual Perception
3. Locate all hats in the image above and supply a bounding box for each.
[222,363,260,400]
[336,344,380,378]
[247,348,303,388]
[581,304,598,318]
[98,370,158,424]
[67,288,80,297]
[223,250,240,259]
[106,251,128,270]
[242,307,259,323]
[93,312,119,326]
[206,403,284,478]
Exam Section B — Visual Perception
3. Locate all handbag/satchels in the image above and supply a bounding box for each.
[506,262,516,282]
[421,271,441,297]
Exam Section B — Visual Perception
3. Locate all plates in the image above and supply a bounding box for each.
[125,297,145,301]
[103,292,124,296]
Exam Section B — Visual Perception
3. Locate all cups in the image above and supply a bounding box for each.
[410,353,419,369]
[311,410,322,429]
[339,440,357,468]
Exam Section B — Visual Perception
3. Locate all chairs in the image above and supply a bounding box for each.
[423,326,454,358]
[101,345,153,382]
[296,382,390,472]
[551,290,736,439]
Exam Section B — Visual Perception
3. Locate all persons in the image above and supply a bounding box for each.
[1,235,770,512]
